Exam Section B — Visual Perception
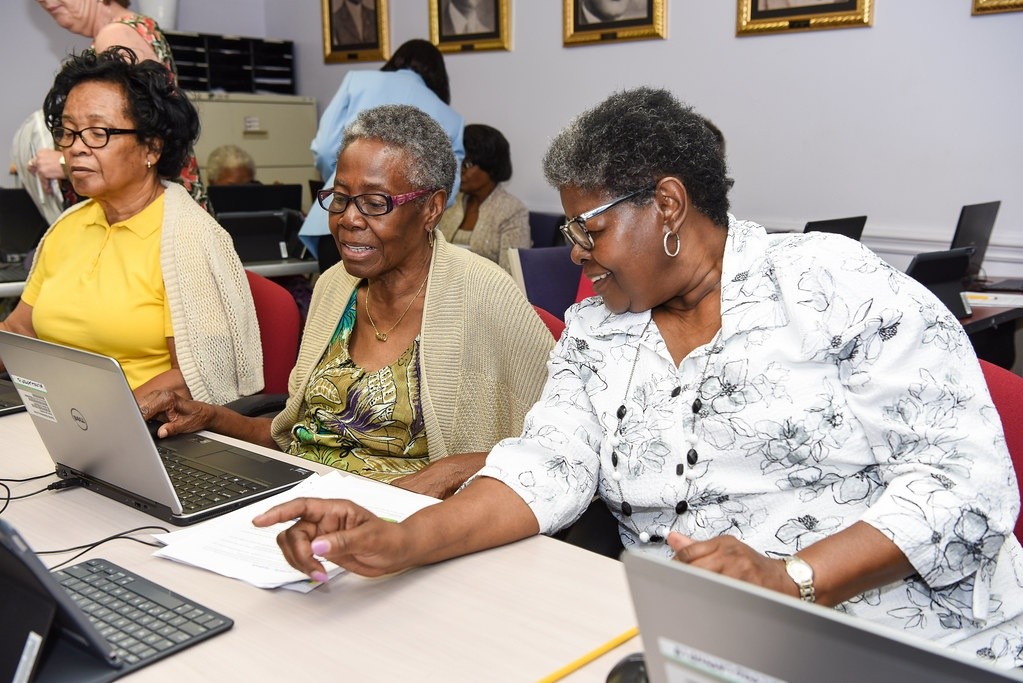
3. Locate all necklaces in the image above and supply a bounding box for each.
[612,314,722,546]
[365,277,428,342]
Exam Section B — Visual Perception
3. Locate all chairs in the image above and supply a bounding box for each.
[247,269,300,396]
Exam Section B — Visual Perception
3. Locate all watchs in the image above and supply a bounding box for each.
[783,555,816,603]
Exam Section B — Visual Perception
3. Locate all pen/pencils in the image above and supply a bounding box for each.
[529,628,640,683]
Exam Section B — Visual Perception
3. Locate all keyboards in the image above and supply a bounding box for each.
[49,557,233,669]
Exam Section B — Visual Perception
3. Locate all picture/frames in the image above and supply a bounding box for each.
[562,1,667,46]
[320,0,390,65]
[735,0,874,38]
[427,0,514,51]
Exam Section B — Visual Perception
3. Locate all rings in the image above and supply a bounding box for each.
[28,159,33,166]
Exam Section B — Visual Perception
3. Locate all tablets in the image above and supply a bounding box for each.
[216,210,287,243]
[905,245,978,282]
[0,516,125,670]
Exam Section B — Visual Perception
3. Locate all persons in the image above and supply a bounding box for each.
[333,0,377,45]
[296,39,467,276]
[206,145,260,186]
[253,87,1023,678]
[136,103,558,500]
[443,0,495,35]
[0,47,266,406]
[436,123,532,276]
[578,0,644,25]
[26,0,216,224]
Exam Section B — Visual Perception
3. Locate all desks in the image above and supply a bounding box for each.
[0,277,1023,683]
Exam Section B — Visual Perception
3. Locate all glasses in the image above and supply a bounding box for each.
[559,176,685,251]
[317,185,446,216]
[462,157,476,169]
[51,125,152,148]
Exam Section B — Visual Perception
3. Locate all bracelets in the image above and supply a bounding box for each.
[60,157,68,178]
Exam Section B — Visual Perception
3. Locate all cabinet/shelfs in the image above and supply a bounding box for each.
[180,91,318,214]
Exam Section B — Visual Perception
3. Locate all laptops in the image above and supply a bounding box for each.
[0,188,50,264]
[0,330,320,525]
[206,183,302,219]
[622,547,1023,683]
[803,216,868,242]
[949,200,1001,282]
